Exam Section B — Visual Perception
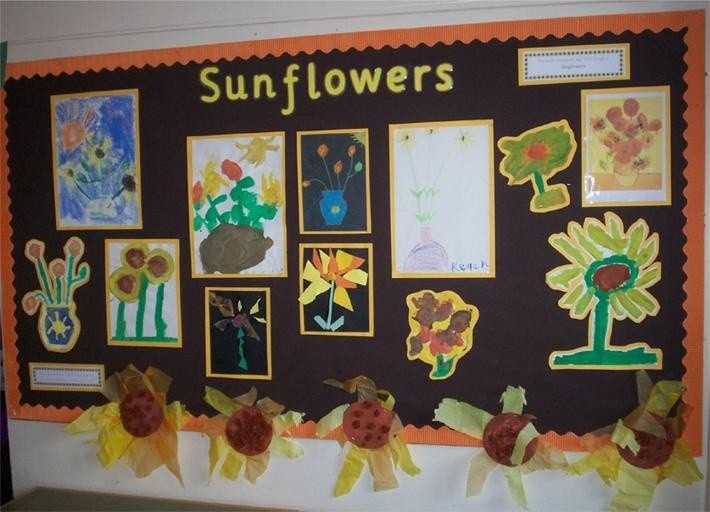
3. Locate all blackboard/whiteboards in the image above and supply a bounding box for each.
[0,2,710,512]
